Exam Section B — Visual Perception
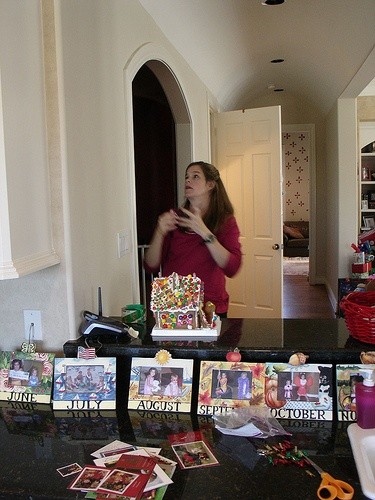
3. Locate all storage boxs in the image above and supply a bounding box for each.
[352,252,372,278]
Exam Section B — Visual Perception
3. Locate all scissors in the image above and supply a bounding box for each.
[302,454,354,500]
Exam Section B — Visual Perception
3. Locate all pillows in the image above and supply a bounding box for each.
[283,226,304,239]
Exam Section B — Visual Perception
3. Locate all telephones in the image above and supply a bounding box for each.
[79,287,127,342]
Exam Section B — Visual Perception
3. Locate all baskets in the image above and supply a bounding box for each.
[339,289,375,344]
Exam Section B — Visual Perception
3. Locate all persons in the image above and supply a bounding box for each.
[296,372,310,401]
[58,368,105,394]
[215,372,228,399]
[143,367,159,395]
[8,359,41,388]
[184,443,209,466]
[283,379,293,401]
[164,373,181,397]
[237,372,250,399]
[61,467,77,474]
[143,162,243,319]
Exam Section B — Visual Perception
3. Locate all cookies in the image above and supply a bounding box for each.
[150,272,209,330]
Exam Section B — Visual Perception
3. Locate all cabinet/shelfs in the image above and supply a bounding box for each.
[358,121,375,235]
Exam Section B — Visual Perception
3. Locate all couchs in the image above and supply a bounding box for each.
[282,221,310,257]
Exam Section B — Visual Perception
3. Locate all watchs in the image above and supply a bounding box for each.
[202,234,217,244]
